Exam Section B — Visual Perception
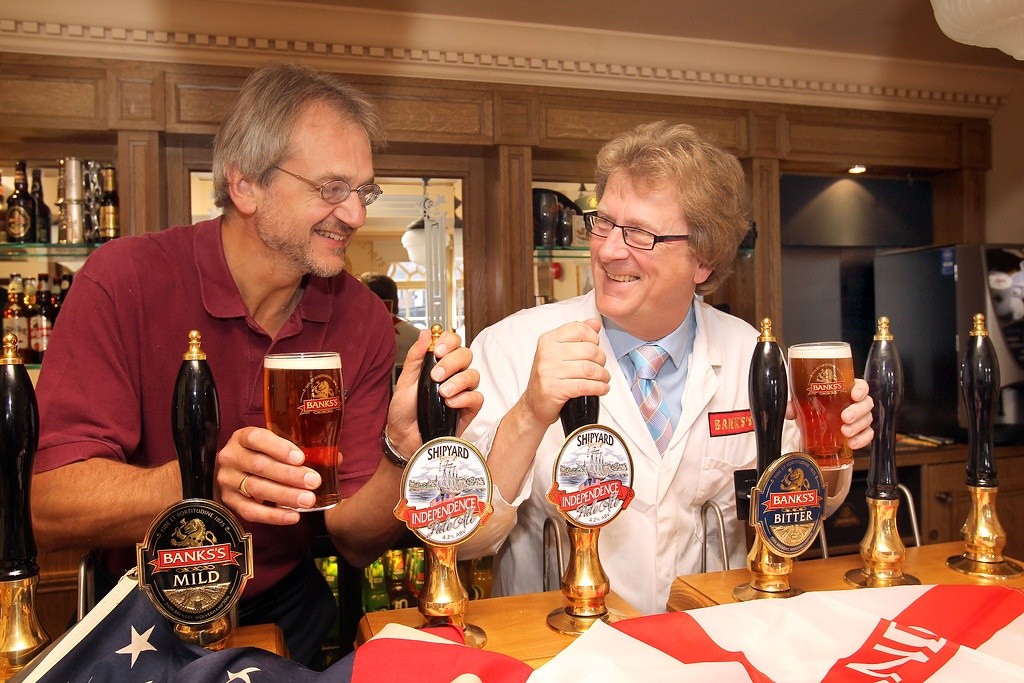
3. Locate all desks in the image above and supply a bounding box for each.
[666,540,1024,683]
[352,588,647,669]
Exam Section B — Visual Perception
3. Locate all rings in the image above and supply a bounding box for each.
[238,475,251,498]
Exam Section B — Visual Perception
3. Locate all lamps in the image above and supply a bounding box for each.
[930,0,1024,61]
[400,177,463,267]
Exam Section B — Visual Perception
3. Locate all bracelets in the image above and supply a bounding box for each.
[381,426,409,468]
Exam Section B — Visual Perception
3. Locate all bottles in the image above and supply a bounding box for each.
[0,157,119,364]
[320,548,425,611]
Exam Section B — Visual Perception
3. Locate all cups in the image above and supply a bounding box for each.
[263,351,342,513]
[787,341,855,472]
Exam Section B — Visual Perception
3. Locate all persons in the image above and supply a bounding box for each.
[28,62,485,666]
[360,272,423,366]
[448,120,875,618]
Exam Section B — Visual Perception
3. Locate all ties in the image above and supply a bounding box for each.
[627,344,673,459]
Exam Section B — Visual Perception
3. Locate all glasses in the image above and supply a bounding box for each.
[273,165,383,207]
[583,210,690,251]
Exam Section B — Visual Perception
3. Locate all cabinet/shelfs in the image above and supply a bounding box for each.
[798,434,1024,562]
[0,242,102,369]
